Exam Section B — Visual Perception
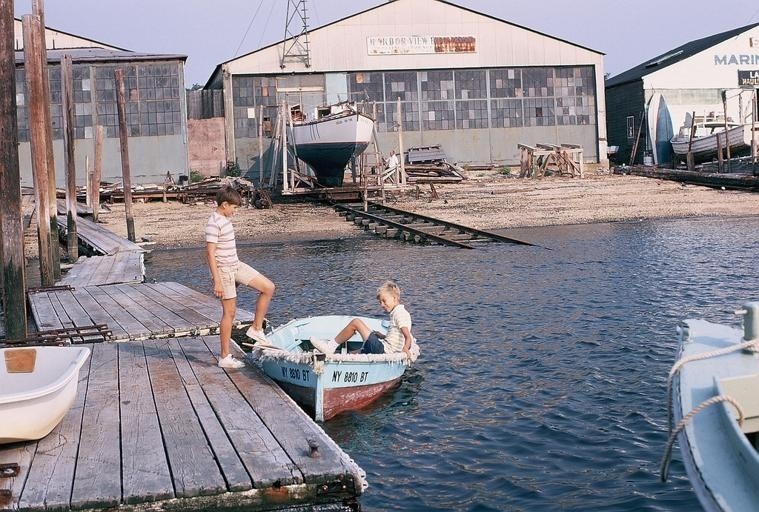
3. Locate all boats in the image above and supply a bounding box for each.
[669,126,759,157]
[0,345,90,443]
[674,303,759,512]
[278,107,375,185]
[252,316,421,420]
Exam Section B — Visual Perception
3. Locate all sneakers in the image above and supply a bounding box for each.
[246,326,272,346]
[309,336,335,354]
[218,354,246,368]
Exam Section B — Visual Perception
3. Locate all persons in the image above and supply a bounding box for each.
[381,149,398,184]
[309,280,412,359]
[205,186,276,369]
